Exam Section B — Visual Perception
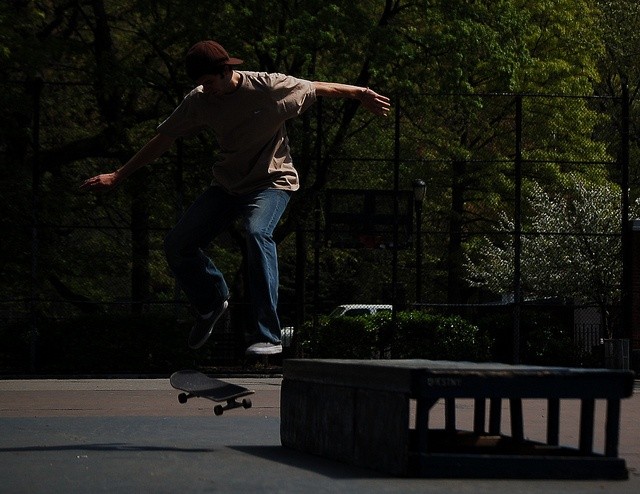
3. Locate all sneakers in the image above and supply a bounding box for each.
[189,301,228,350]
[245,342,284,355]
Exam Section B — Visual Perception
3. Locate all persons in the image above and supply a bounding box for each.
[78,41,390,358]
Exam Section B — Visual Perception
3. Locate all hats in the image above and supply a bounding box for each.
[185,40,244,68]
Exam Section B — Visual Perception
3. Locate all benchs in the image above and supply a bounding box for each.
[277,356,635,484]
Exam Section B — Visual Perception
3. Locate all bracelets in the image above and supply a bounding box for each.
[365,87,369,94]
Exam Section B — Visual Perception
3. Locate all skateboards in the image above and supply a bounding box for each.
[169,366,255,416]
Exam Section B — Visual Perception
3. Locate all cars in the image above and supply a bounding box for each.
[281,303,396,347]
